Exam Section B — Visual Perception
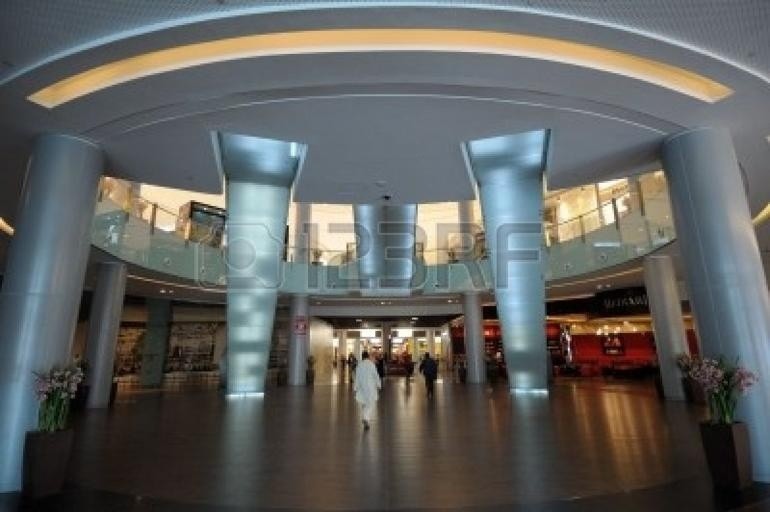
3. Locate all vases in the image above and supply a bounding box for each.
[699,420,752,490]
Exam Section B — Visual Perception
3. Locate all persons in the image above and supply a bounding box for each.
[605,336,622,348]
[348,349,386,432]
[487,358,506,392]
[407,360,413,378]
[420,352,437,400]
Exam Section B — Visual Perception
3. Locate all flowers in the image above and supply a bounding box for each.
[678,352,759,425]
[35,364,84,432]
